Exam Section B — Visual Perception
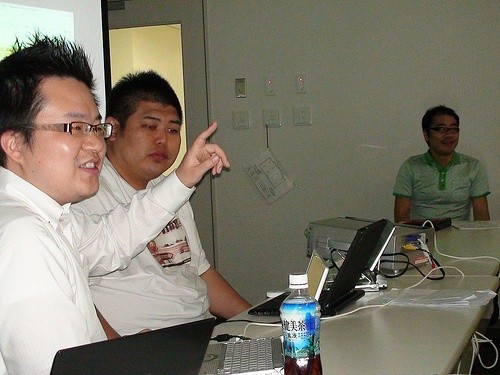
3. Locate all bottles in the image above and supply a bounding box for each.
[280,272,322,375]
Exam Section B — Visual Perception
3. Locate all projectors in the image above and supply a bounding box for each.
[306,216,435,274]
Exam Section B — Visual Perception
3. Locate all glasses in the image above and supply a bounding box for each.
[426,126,460,134]
[8,121,113,139]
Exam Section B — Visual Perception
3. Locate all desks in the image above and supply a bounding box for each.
[197,219,500,375]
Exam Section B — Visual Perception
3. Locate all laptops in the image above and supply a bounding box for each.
[49,218,396,375]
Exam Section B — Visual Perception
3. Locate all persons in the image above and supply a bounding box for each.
[72,71,253,339]
[393,105,490,224]
[0,36,231,375]
